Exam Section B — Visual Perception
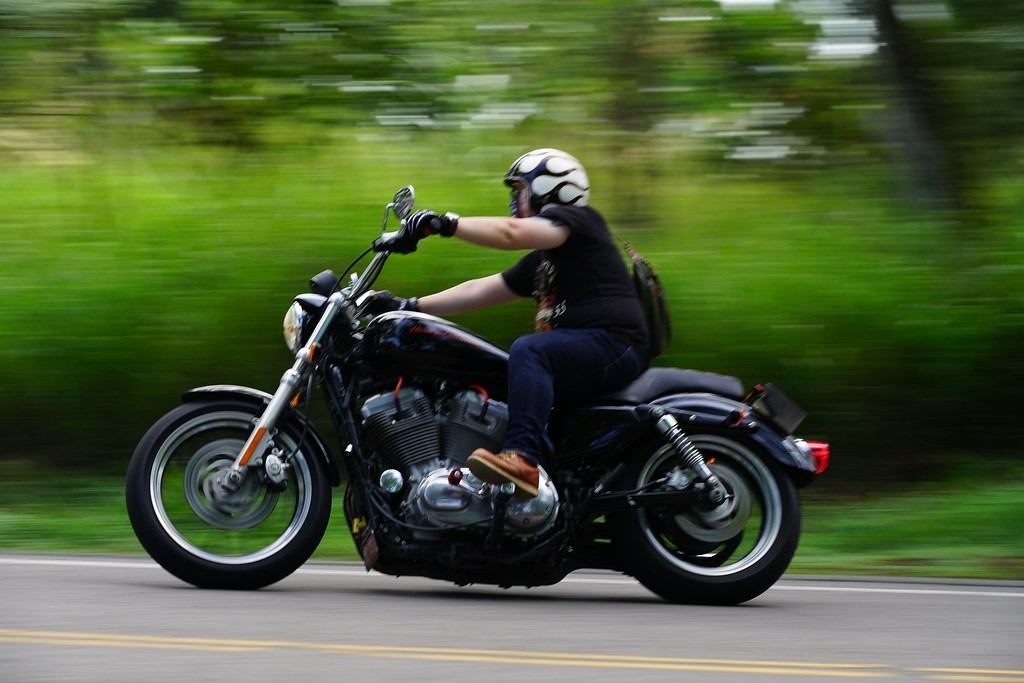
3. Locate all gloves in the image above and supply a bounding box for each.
[369,289,420,318]
[407,208,460,239]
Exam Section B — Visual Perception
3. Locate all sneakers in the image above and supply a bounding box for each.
[467,447,540,498]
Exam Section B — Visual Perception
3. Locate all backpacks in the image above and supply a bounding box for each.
[625,240,672,360]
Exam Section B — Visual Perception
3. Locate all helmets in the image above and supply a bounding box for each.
[503,147,591,218]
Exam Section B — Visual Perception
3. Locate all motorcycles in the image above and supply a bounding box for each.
[125,185,830,605]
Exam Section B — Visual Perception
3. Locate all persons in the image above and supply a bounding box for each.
[357,147,651,498]
